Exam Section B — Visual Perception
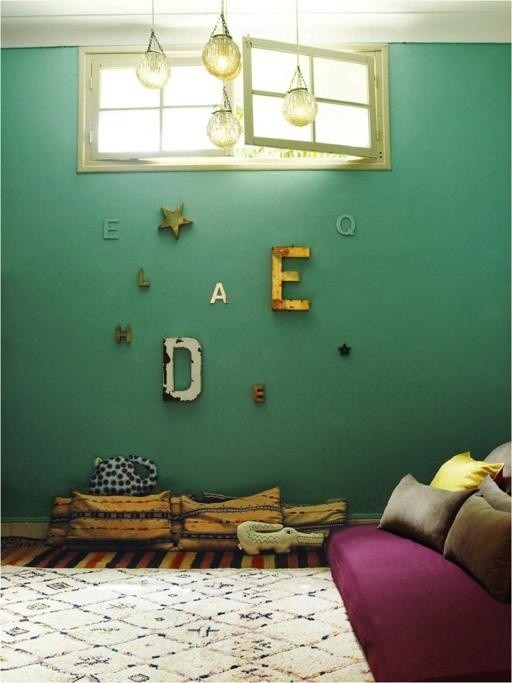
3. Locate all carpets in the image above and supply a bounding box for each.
[1,566,376,683]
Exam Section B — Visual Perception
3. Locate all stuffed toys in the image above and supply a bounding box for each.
[88,451,163,499]
[236,517,325,558]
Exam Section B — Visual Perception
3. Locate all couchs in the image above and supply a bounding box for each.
[326,524,512,683]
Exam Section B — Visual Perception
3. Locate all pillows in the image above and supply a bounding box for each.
[280,499,346,528]
[67,489,175,552]
[48,494,182,545]
[378,441,512,603]
[176,486,286,541]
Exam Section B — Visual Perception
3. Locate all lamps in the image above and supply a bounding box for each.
[280,1,318,124]
[202,0,242,82]
[134,3,171,89]
[206,86,242,147]
